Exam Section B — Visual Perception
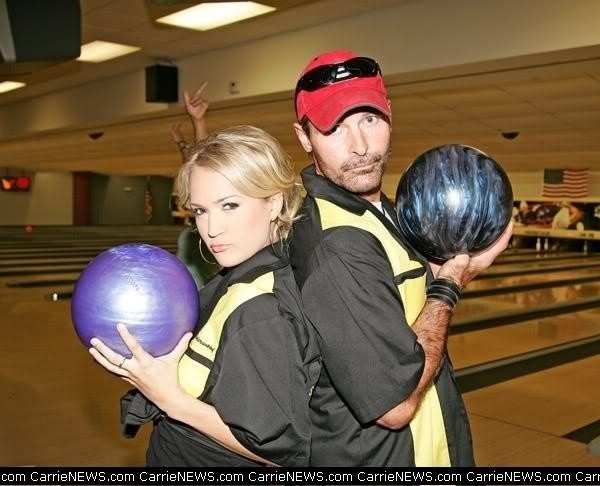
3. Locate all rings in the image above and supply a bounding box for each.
[116,358,128,369]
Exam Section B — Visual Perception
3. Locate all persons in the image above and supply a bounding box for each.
[287,47,514,470]
[88,125,322,470]
[167,79,224,291]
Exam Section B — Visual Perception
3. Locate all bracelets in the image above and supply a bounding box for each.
[425,278,463,311]
[180,144,193,150]
[176,140,186,144]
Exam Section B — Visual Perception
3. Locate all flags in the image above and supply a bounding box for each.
[543,166,589,199]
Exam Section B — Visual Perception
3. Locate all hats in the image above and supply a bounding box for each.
[295,50,393,136]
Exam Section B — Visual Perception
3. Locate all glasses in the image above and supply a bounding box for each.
[294,57,382,123]
